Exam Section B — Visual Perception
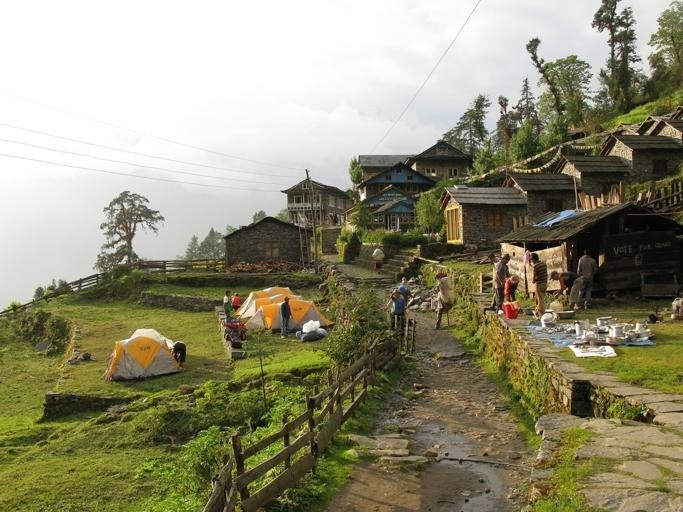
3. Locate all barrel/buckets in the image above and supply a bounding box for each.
[504,303,518,319]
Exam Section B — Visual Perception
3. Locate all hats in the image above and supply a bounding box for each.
[400,287,408,293]
[549,271,557,280]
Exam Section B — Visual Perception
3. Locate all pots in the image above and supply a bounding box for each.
[596,317,631,337]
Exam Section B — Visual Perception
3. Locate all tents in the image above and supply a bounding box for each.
[239,293,303,319]
[237,286,294,315]
[104,328,186,381]
[244,300,335,334]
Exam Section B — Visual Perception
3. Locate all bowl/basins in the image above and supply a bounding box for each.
[523,308,575,319]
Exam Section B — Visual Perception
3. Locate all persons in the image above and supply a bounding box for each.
[529,253,548,320]
[577,249,599,310]
[231,293,243,312]
[434,273,455,329]
[549,271,583,310]
[488,254,519,314]
[172,341,186,367]
[223,290,233,318]
[280,297,293,339]
[384,277,415,337]
[372,247,385,275]
